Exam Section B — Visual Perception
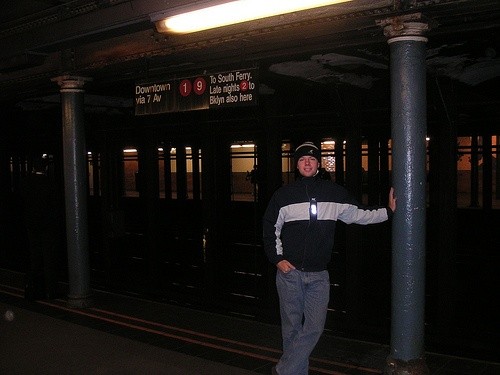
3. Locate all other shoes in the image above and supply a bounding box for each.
[272,366,278,375]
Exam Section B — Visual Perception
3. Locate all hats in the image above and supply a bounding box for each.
[295,141,321,166]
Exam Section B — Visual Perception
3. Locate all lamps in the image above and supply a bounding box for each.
[150,0,354,37]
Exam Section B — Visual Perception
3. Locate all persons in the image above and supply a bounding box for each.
[250,164,259,196]
[261,141,396,375]
